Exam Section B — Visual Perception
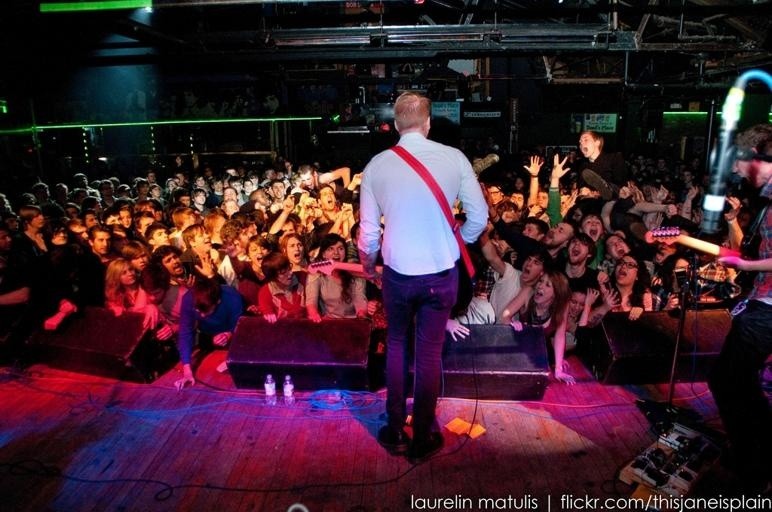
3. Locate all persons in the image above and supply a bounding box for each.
[357,88,491,466]
[705,124,771,512]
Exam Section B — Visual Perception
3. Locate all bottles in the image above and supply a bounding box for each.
[264,374,276,405]
[281,377,296,408]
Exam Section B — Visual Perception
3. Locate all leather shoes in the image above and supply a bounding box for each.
[377,423,410,455]
[406,431,445,465]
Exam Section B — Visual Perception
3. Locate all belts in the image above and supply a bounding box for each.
[383,265,453,281]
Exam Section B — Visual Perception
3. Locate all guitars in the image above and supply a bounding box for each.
[645,227,759,294]
[308,258,477,319]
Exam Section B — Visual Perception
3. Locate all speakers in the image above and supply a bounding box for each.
[406,322,549,401]
[575,308,736,385]
[34,305,182,384]
[224,316,389,393]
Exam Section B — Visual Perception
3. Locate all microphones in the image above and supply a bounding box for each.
[699,86,746,233]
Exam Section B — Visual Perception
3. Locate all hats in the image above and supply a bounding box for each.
[291,186,307,195]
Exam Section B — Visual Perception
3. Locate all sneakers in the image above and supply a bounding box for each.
[581,168,613,201]
